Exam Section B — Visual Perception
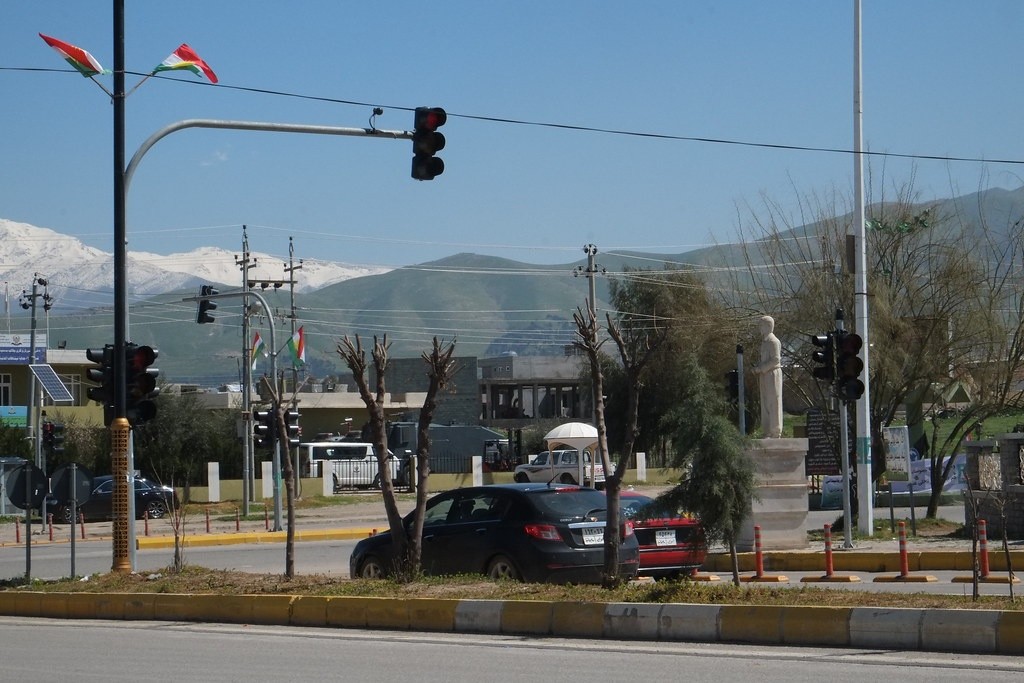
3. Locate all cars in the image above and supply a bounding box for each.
[349,483,641,587]
[600,490,709,578]
[40,475,180,524]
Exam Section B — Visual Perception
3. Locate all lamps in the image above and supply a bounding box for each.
[58,340,66,350]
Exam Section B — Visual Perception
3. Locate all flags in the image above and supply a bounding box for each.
[287,325,306,368]
[39,32,113,78]
[151,43,218,84]
[251,331,266,371]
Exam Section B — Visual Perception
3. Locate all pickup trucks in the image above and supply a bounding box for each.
[513,448,617,489]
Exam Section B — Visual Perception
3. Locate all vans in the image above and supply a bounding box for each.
[297,442,401,494]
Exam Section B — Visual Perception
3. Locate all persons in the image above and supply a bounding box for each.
[752,316,783,438]
[459,498,475,520]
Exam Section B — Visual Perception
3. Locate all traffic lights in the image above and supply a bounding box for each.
[44,421,52,450]
[812,332,835,384]
[834,331,864,403]
[53,424,65,454]
[85,344,116,426]
[254,409,276,450]
[411,105,448,181]
[195,284,219,324]
[284,411,301,449]
[123,344,157,425]
[145,345,161,399]
